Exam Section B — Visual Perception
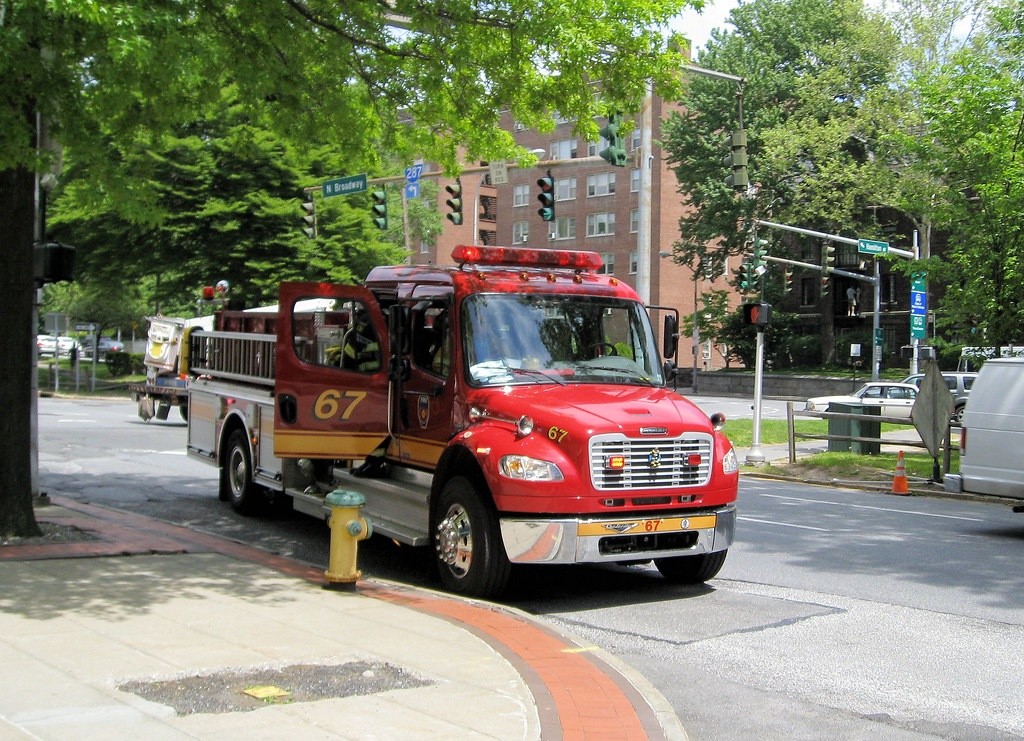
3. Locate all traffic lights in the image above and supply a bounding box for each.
[370,191,389,230]
[742,303,769,327]
[443,181,465,226]
[598,108,628,169]
[299,202,317,239]
[875,327,884,346]
[900,345,913,359]
[739,263,751,295]
[783,269,794,298]
[750,265,760,290]
[536,175,555,222]
[820,245,836,277]
[920,347,934,361]
[721,128,749,194]
[820,275,830,299]
[752,237,768,270]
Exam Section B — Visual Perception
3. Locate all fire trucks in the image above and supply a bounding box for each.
[179,245,741,597]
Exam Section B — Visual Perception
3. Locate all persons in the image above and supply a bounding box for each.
[339,294,387,477]
[847,285,860,316]
[434,312,512,376]
[68,343,76,369]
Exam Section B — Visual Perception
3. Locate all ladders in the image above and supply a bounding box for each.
[179,328,306,388]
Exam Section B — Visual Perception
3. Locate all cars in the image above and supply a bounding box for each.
[805,381,919,418]
[36,333,124,361]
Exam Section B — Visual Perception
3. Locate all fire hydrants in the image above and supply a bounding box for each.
[318,488,374,592]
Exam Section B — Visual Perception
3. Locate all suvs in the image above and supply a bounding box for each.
[941,358,1024,516]
[866,371,978,427]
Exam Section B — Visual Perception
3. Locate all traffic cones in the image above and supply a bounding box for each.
[885,451,913,497]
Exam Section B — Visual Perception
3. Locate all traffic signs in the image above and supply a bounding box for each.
[74,323,98,332]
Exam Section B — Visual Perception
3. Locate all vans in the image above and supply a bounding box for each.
[956,346,1024,372]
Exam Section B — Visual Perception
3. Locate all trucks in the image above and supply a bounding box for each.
[127,296,336,424]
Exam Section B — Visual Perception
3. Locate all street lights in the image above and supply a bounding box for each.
[475,147,546,247]
[657,250,699,394]
